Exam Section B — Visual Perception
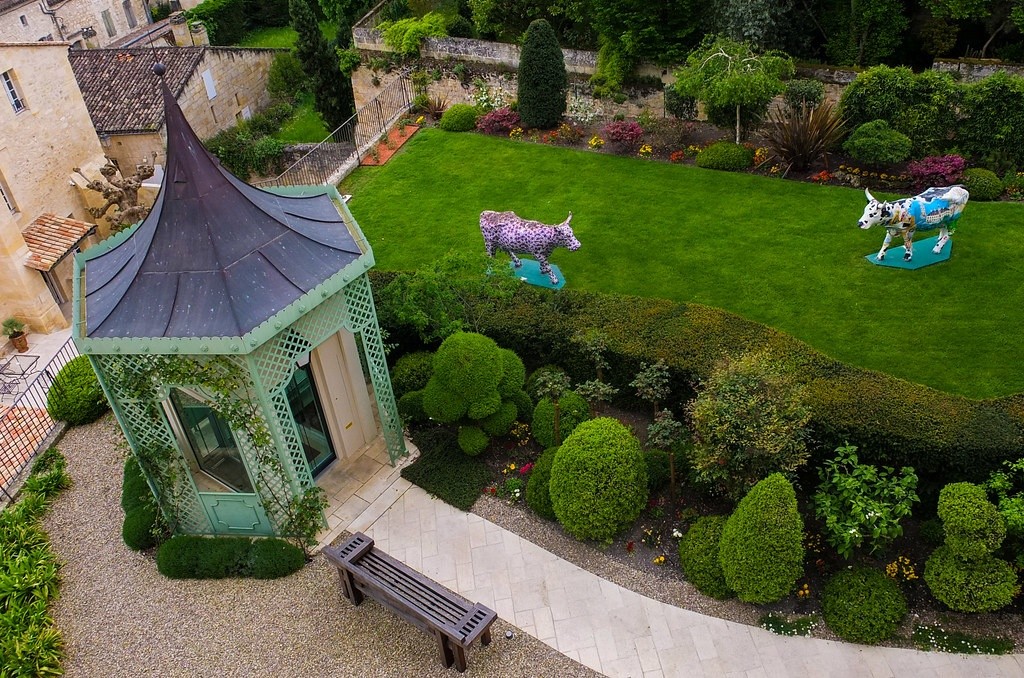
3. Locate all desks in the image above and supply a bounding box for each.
[0,355,44,388]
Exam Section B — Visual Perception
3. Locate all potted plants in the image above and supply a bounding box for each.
[420,89,453,120]
[2,318,29,353]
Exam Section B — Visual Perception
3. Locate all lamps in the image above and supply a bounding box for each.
[99,132,111,152]
[51,16,68,35]
[282,328,313,371]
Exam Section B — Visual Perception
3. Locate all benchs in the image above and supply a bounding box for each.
[322,531,496,674]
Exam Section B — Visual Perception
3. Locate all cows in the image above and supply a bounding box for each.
[857,184,969,261]
[479,210,581,284]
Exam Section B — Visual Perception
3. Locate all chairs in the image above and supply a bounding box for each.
[0,358,15,377]
[0,378,24,403]
